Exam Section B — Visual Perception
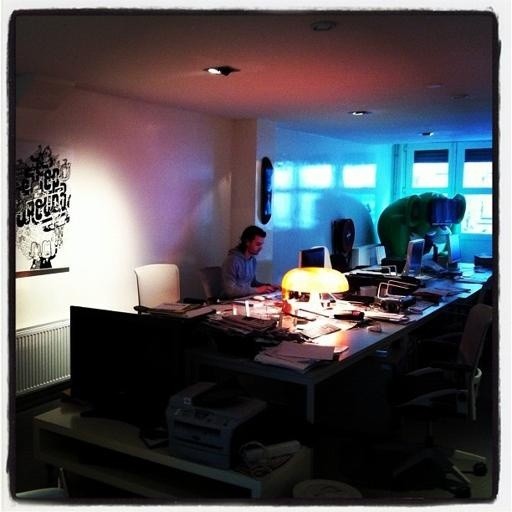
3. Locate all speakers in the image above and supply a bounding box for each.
[331,218,355,270]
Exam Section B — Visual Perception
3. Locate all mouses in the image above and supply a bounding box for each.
[250,295,265,301]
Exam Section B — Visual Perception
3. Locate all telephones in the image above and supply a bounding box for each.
[421,260,443,273]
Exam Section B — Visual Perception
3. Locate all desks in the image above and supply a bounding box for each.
[32,404,312,504]
[187,268,483,450]
[362,260,494,284]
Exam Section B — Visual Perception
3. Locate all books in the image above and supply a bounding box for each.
[148,303,277,337]
[260,342,334,374]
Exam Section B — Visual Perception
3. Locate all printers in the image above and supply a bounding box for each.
[168,380,268,469]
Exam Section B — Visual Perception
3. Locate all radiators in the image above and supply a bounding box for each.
[15,320,71,400]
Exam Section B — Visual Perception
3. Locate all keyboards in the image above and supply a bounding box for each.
[261,289,282,299]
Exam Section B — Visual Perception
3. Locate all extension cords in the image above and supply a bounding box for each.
[241,439,303,463]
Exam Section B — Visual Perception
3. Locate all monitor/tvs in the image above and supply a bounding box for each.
[402,238,425,278]
[298,247,325,307]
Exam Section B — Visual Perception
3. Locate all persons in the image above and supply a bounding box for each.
[218,225,282,300]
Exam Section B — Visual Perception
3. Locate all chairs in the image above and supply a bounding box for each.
[134,263,207,314]
[201,264,229,305]
[357,303,494,495]
